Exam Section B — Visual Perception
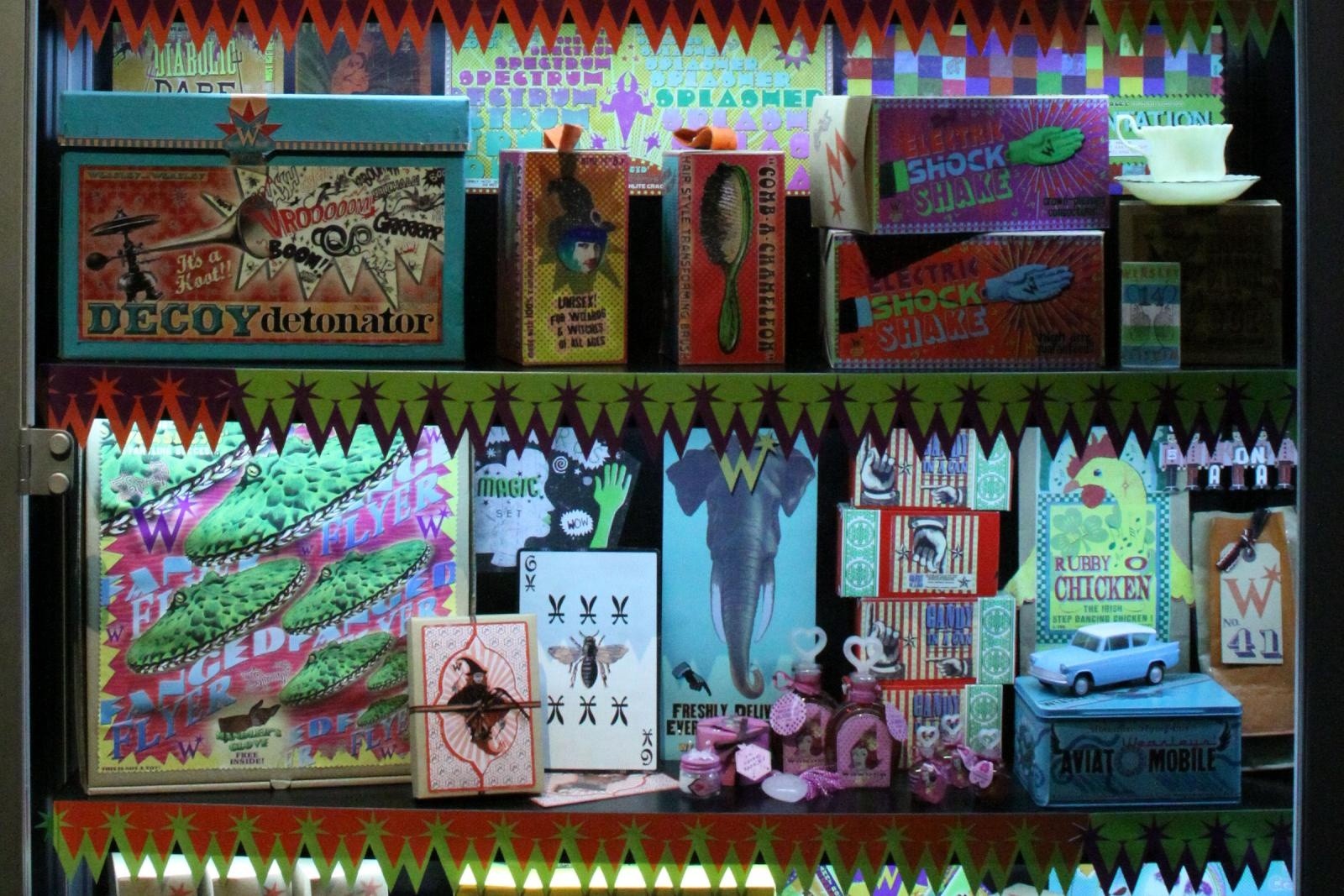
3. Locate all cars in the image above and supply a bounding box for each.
[1027,621,1180,698]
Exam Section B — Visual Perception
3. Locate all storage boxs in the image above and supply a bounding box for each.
[1013,674,1244,807]
[56,92,1121,373]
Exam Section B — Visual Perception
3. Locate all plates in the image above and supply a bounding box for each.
[1114,174,1261,205]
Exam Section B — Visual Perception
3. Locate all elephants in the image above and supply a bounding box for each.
[669,433,814,704]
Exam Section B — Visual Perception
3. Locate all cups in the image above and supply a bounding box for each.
[1116,115,1234,178]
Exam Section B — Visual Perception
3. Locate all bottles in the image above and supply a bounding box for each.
[824,673,902,799]
[771,662,837,776]
[679,751,721,799]
